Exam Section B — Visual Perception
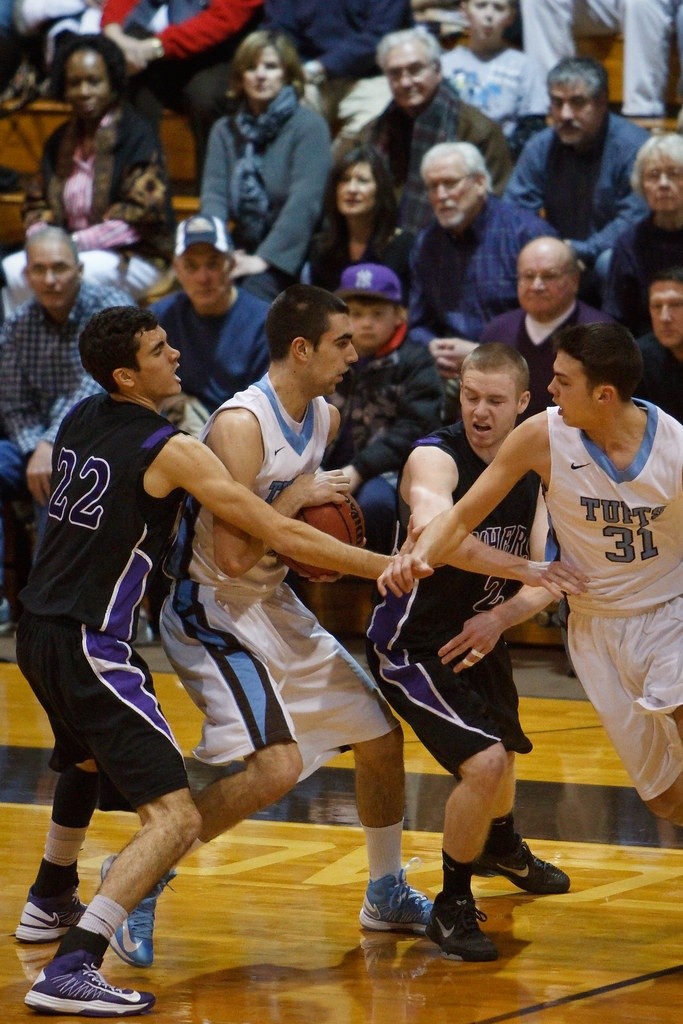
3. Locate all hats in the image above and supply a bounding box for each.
[331,263,402,301]
[171,214,232,257]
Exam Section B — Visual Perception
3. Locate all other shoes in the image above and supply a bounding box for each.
[129,616,154,645]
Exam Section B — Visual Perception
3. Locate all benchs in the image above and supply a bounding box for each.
[0,31,683,646]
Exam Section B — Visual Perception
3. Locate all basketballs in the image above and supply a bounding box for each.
[277,492,365,580]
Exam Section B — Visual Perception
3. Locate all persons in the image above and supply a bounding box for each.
[331,263,446,541]
[364,342,571,962]
[632,265,683,425]
[501,55,652,314]
[146,215,272,438]
[41,34,177,264]
[303,144,415,293]
[0,226,137,632]
[478,236,615,428]
[377,315,683,827]
[252,0,414,166]
[101,282,434,965]
[600,134,683,340]
[12,305,396,1017]
[199,30,330,297]
[441,0,550,149]
[99,0,265,157]
[405,141,559,379]
[520,0,680,119]
[356,27,514,235]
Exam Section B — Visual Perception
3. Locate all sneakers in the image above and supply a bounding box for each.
[471,832,571,894]
[359,857,434,935]
[15,882,88,942]
[425,892,498,961]
[101,855,177,965]
[24,950,156,1018]
